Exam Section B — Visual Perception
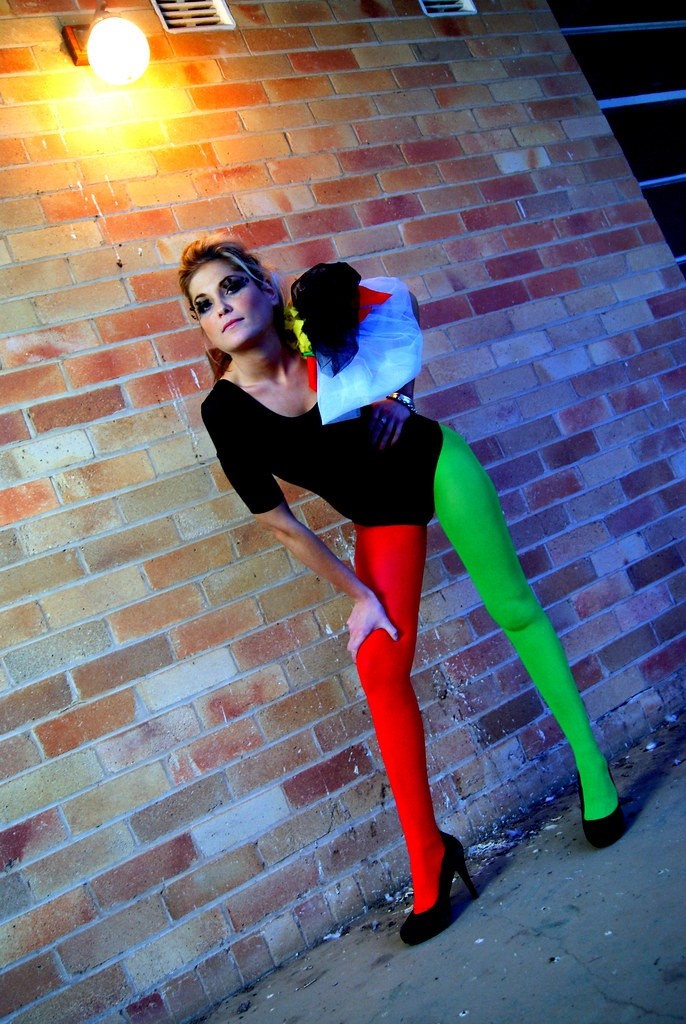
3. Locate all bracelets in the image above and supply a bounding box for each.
[385,392,417,414]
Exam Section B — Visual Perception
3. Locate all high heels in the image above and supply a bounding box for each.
[400,830,479,946]
[576,765,626,849]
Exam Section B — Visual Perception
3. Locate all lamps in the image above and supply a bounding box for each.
[61,1,151,86]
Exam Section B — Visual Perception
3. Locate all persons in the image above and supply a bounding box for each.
[176,240,623,946]
